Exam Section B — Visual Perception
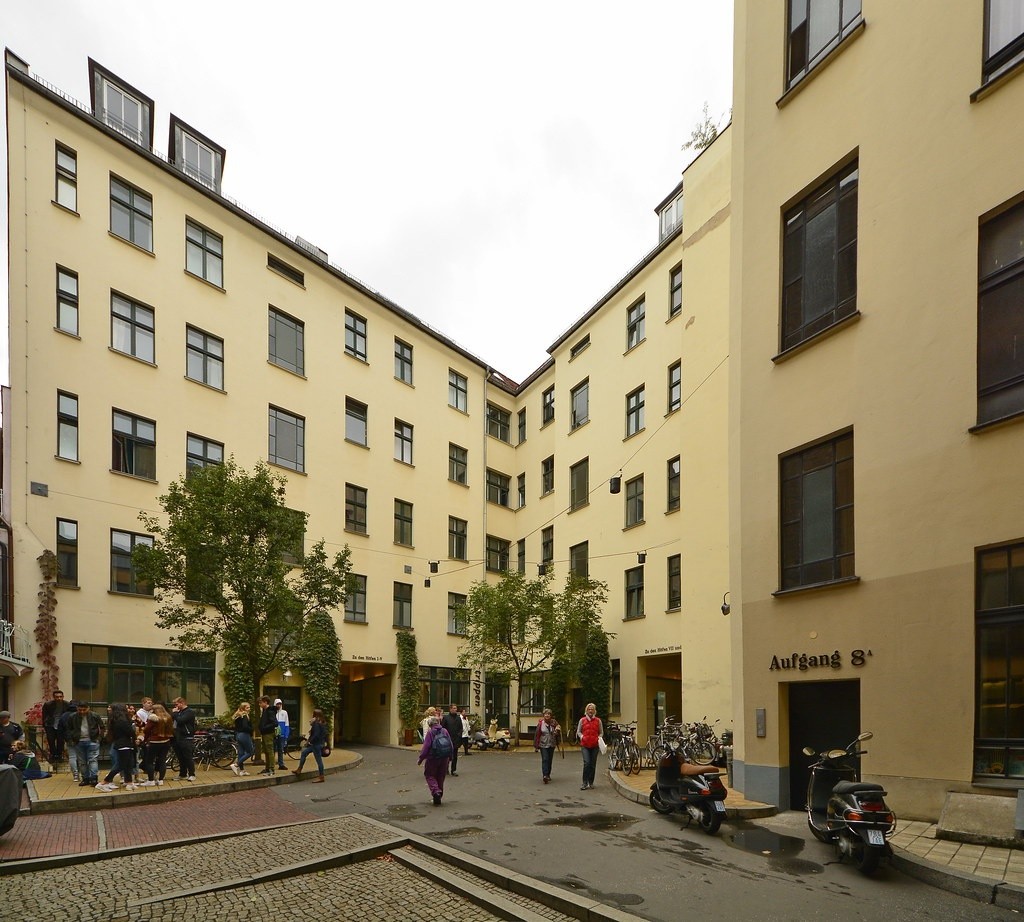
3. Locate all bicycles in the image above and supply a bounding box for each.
[602,714,720,777]
[566,713,586,746]
[250,724,308,762]
[153,717,246,772]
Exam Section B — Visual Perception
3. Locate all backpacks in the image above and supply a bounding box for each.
[429,728,451,760]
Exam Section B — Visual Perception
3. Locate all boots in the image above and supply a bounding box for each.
[312,775,325,783]
[292,766,302,776]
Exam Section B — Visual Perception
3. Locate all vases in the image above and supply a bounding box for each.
[405,729,414,746]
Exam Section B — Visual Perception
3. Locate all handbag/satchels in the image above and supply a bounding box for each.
[597,736,608,755]
[321,736,331,757]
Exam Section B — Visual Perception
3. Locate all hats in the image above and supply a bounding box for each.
[0,711,11,717]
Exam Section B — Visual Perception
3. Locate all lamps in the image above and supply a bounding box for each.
[424,578,431,588]
[428,560,440,573]
[609,471,623,494]
[283,669,292,684]
[637,550,647,564]
[721,592,732,616]
[538,564,547,575]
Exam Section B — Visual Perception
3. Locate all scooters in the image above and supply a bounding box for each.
[803,731,897,874]
[484,710,512,751]
[465,712,489,751]
[649,723,728,836]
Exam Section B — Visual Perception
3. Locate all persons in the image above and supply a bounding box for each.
[257,695,278,777]
[0,691,197,793]
[230,702,254,777]
[420,704,472,777]
[417,716,454,806]
[577,703,603,790]
[291,709,325,783]
[534,708,561,784]
[274,699,289,771]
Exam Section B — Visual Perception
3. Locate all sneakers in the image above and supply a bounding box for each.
[95,774,149,793]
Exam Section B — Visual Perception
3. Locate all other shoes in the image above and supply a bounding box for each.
[89,777,97,787]
[433,793,442,807]
[544,776,548,784]
[74,779,78,782]
[589,784,594,789]
[581,783,588,790]
[230,764,239,776]
[450,770,458,777]
[140,779,155,787]
[188,776,196,781]
[465,752,472,755]
[548,775,551,781]
[240,771,250,776]
[157,779,164,786]
[172,775,187,782]
[79,779,88,786]
[279,765,288,770]
[261,768,275,775]
